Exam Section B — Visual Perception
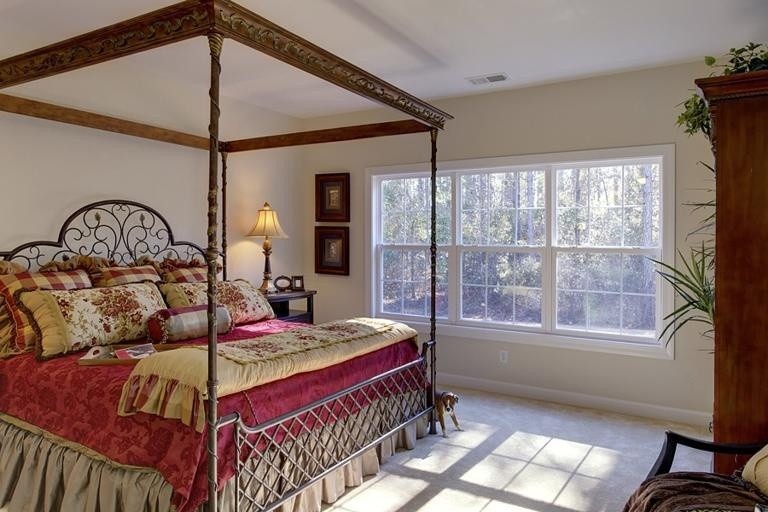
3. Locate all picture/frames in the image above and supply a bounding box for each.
[315,172,350,223]
[314,226,350,276]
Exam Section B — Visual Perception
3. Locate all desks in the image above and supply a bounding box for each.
[264,290,317,324]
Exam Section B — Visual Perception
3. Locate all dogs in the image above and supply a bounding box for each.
[434,391,465,438]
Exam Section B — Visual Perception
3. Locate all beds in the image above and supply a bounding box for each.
[0,200,432,511]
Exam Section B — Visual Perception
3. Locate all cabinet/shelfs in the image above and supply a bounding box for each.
[696,70,768,477]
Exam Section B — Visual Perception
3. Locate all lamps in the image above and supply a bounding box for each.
[242,202,289,293]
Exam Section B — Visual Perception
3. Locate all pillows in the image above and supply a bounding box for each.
[1,258,275,361]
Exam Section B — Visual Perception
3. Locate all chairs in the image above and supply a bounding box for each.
[623,430,768,511]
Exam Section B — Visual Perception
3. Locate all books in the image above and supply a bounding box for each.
[95,342,159,360]
